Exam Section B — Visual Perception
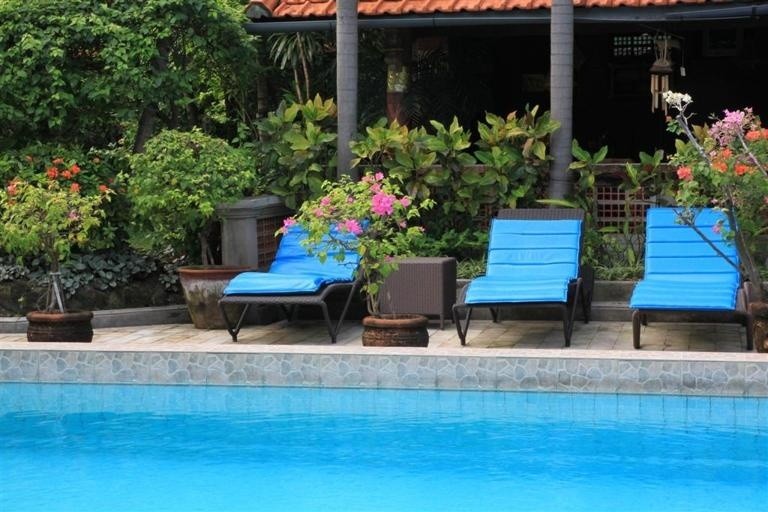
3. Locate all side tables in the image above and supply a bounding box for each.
[376,256,456,329]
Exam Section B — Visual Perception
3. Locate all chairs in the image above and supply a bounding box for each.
[218,217,373,343]
[629,207,754,351]
[450,208,590,348]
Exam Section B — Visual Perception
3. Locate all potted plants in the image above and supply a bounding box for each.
[127,124,259,331]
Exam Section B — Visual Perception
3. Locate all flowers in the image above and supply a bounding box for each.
[660,90,768,301]
[273,171,438,318]
[0,158,119,312]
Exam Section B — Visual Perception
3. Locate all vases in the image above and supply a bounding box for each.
[747,303,768,353]
[26,308,94,343]
[361,313,430,348]
